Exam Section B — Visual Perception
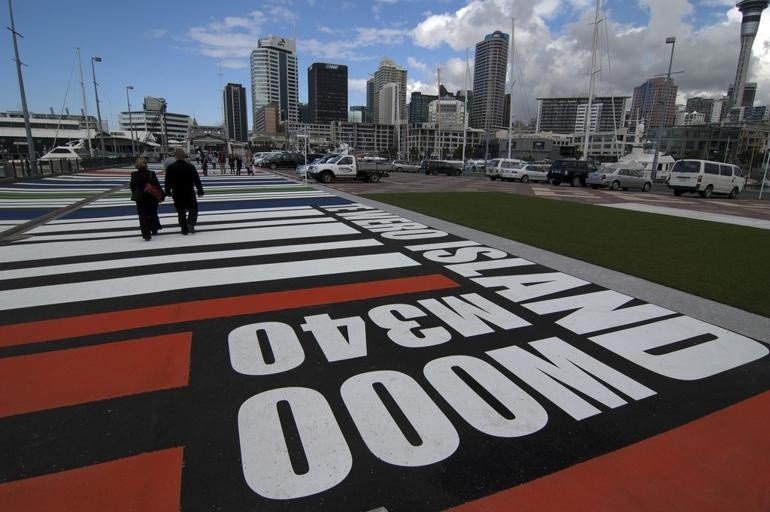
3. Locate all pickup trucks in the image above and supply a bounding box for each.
[295,153,396,183]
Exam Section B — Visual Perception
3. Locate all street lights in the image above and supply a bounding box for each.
[91,56,107,151]
[652,37,676,184]
[126,85,136,155]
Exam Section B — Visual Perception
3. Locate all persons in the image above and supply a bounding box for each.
[164,148,205,236]
[191,149,257,176]
[129,157,166,242]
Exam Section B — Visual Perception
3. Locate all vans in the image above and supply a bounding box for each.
[423,160,462,176]
[667,158,745,199]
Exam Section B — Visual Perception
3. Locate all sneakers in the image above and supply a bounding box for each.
[145,225,196,241]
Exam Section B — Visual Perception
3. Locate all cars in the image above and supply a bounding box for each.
[486,157,652,190]
[391,159,422,172]
[253,151,301,170]
[40,146,89,165]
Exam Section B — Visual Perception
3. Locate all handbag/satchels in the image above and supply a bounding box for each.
[144,183,164,202]
